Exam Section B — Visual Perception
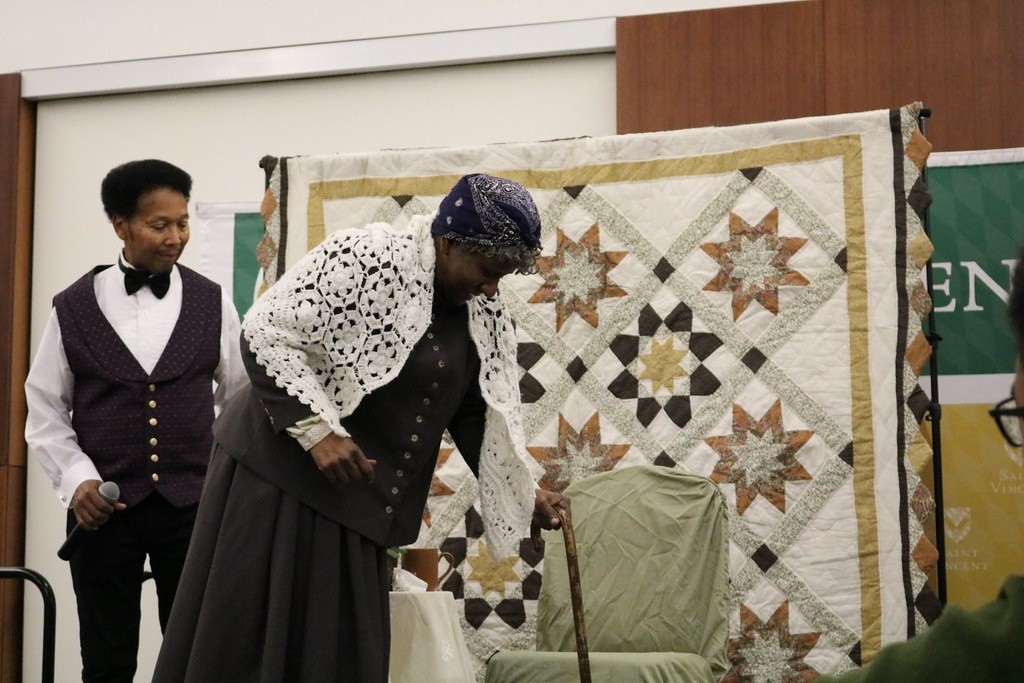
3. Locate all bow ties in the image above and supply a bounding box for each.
[118,254,171,300]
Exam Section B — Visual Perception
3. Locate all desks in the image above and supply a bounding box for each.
[387,591,476,683]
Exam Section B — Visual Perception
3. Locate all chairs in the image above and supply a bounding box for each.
[484,464,730,683]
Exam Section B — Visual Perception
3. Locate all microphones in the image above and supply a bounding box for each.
[57,482,120,561]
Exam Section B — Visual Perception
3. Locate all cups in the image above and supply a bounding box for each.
[401,548,454,591]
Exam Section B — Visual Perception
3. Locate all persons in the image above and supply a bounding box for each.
[25,159,251,682]
[150,174,571,682]
[810,253,1024,683]
